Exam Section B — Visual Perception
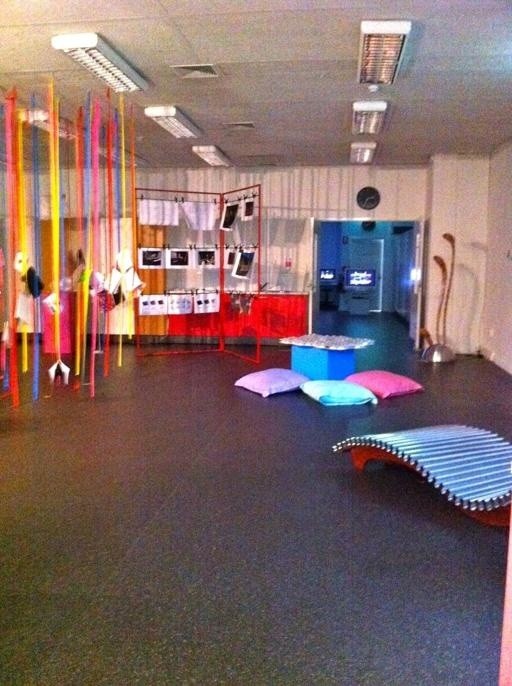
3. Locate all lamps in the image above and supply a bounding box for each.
[346,102,388,164]
[144,106,203,139]
[50,32,149,101]
[189,145,233,168]
[356,18,412,88]
[423,232,457,365]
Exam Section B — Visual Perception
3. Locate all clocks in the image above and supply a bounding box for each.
[356,186,383,211]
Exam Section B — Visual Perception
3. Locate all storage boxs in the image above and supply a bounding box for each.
[290,342,355,379]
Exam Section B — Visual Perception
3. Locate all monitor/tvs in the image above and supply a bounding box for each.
[343,266,377,292]
[319,268,336,283]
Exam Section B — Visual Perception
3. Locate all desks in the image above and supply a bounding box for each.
[162,289,315,348]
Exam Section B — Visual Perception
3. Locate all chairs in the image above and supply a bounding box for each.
[332,422,510,534]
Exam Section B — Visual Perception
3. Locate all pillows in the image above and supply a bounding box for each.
[234,364,423,411]
